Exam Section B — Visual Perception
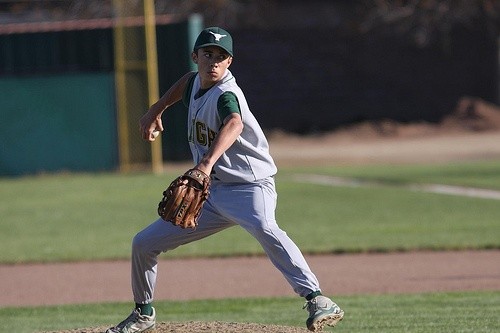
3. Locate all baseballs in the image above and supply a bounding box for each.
[152,130,158,137]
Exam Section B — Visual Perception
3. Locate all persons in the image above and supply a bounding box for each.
[100,25,345,332]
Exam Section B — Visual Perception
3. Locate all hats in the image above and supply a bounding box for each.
[194,26,234,57]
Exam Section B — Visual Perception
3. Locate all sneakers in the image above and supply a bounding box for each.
[105,306,155,333]
[306,295,344,332]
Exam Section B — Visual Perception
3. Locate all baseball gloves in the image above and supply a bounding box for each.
[158,169,211,231]
